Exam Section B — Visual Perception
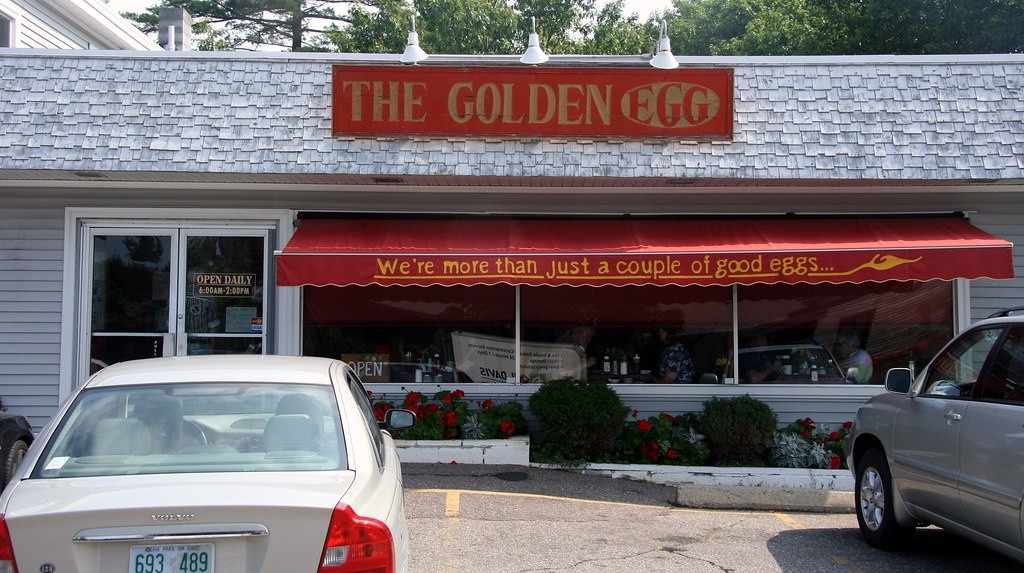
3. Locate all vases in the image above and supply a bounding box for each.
[721,374,727,384]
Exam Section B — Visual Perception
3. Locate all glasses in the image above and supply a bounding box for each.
[834,341,847,348]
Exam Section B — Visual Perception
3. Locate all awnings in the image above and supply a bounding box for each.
[272,206,1016,289]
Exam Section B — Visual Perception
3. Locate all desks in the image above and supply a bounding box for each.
[589,370,651,382]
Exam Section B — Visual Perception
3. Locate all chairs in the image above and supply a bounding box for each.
[89,395,183,457]
[263,393,325,452]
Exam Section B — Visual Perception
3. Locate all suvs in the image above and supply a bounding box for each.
[845,305,1024,563]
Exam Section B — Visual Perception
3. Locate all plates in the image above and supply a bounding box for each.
[609,380,619,383]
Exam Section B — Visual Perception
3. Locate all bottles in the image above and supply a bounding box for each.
[603,347,641,375]
[404,351,454,383]
[776,354,827,381]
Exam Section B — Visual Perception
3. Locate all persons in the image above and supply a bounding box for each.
[833,332,873,383]
[643,327,695,384]
[160,267,221,355]
[554,325,596,368]
[416,329,447,383]
[748,333,782,383]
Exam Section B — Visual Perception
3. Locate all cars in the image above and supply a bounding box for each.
[0,355,418,573]
[0,408,37,494]
[713,342,859,385]
[357,359,476,384]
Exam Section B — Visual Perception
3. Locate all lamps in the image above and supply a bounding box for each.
[520,17,548,65]
[400,15,428,65]
[641,19,679,70]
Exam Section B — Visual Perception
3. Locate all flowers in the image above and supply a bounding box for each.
[715,358,727,374]
[797,347,810,364]
